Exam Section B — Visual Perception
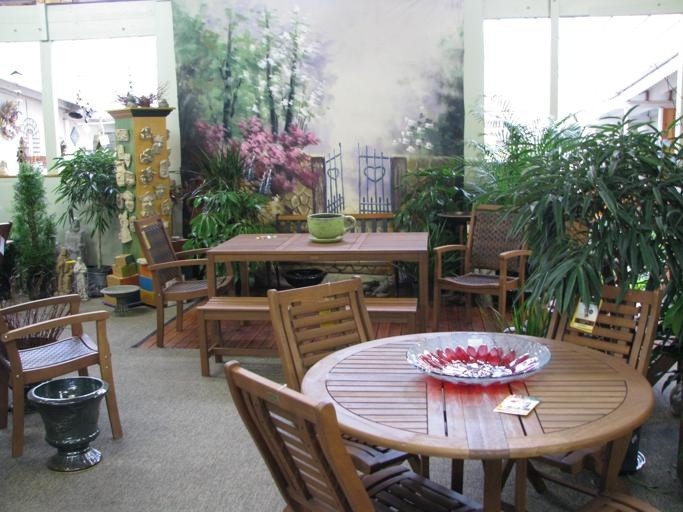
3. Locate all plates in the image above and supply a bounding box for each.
[309,238,344,244]
[403,332,552,388]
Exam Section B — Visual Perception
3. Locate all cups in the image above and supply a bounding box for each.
[305,212,355,239]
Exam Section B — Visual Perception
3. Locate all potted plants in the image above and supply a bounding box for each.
[9,160,56,301]
[127,98,137,109]
[47,143,122,296]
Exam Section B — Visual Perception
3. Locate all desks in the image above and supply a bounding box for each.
[205,232,432,331]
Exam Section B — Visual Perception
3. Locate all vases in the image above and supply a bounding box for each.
[141,104,150,107]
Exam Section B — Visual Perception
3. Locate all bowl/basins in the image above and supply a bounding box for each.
[282,268,326,287]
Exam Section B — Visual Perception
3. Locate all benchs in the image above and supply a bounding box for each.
[275,213,399,296]
[197,296,418,377]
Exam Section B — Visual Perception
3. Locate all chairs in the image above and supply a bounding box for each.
[433,203,534,330]
[133,214,251,347]
[0,294,124,458]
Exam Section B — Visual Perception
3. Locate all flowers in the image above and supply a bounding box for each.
[195,114,322,199]
[115,81,167,106]
